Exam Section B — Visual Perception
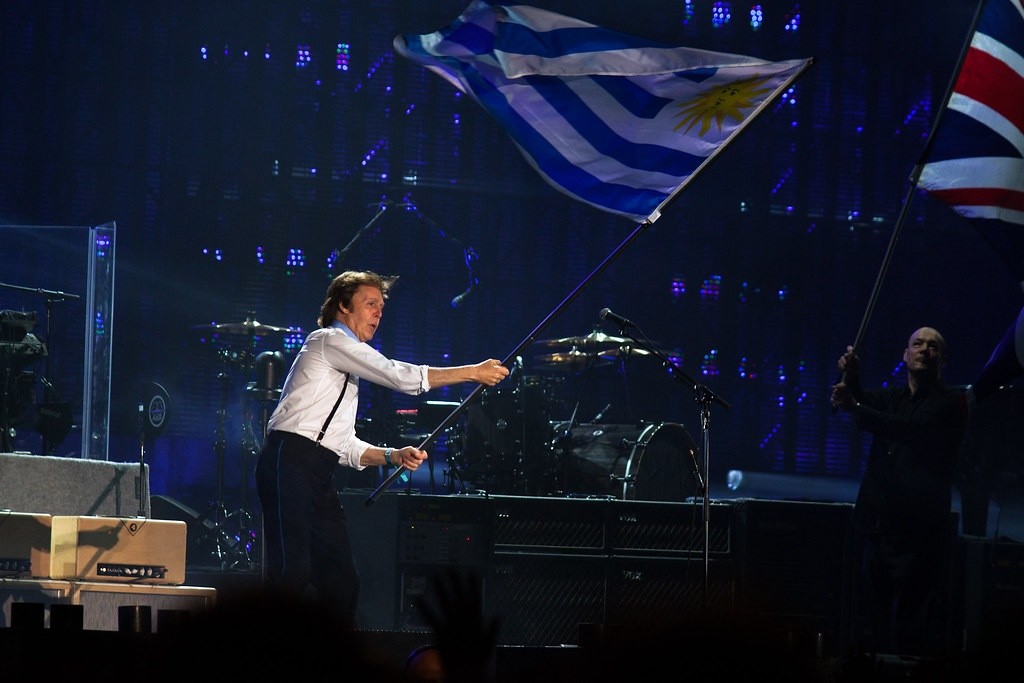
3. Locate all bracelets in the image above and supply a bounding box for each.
[385,447,395,466]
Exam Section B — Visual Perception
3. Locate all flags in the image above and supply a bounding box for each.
[914,0,1023,412]
[392,0,813,227]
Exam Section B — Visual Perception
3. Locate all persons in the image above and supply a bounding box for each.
[256,270,509,629]
[832,327,968,657]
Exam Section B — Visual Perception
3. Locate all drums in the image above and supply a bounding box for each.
[478,373,579,493]
[544,417,706,502]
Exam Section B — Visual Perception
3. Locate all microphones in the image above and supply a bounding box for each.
[599,308,634,327]
[592,402,614,424]
[255,350,280,410]
[509,365,519,381]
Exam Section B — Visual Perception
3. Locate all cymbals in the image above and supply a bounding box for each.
[185,322,303,337]
[530,332,662,368]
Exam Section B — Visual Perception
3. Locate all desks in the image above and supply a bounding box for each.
[0,578,217,610]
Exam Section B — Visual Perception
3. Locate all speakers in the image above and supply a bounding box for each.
[481,497,854,654]
